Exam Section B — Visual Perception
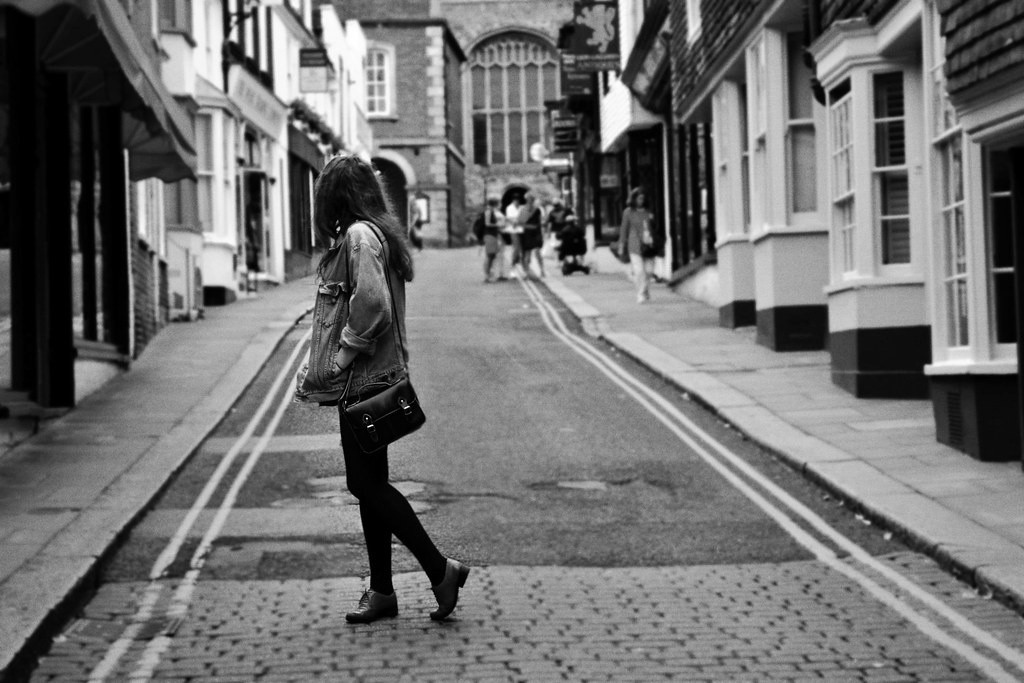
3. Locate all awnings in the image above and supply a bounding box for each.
[0,0,198,187]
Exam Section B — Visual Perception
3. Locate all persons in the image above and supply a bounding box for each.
[408,194,424,253]
[291,154,466,629]
[618,186,659,302]
[481,190,598,283]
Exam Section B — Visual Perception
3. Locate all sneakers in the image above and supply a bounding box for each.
[346,590,399,624]
[430,558,471,620]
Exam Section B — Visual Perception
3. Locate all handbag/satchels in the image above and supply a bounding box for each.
[342,381,425,449]
[641,220,654,245]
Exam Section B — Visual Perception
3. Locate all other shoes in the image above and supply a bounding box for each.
[486,275,492,282]
[637,293,649,304]
[497,276,508,281]
